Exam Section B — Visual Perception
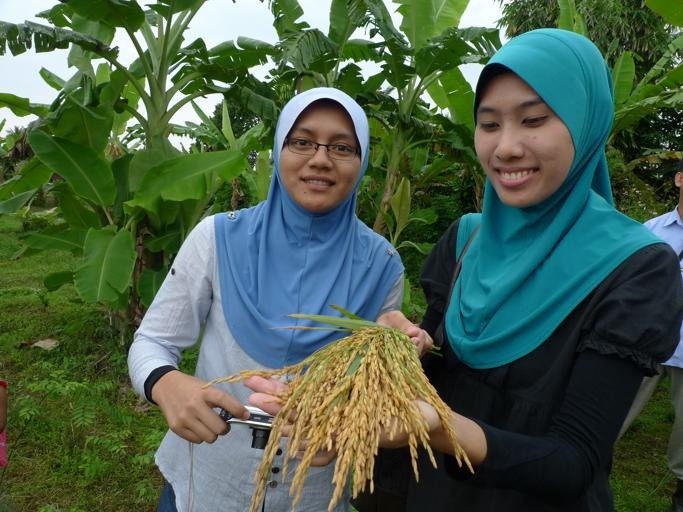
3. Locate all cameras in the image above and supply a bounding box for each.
[219,404,276,449]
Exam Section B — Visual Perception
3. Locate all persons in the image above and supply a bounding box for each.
[613,159,682,510]
[123,86,410,512]
[241,26,683,512]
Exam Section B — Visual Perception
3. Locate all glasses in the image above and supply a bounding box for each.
[285,137,360,161]
